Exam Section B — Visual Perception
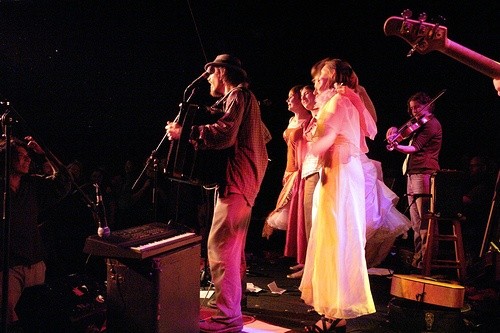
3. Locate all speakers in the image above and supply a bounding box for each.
[429,170,463,215]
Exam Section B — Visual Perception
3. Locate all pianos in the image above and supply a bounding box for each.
[84,221,202,333]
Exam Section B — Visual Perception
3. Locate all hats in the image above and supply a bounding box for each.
[205,54,248,77]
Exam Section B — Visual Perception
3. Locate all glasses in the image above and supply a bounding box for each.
[209,67,215,74]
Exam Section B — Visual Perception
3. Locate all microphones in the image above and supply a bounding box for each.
[403,193,432,199]
[185,65,215,92]
[96,184,110,238]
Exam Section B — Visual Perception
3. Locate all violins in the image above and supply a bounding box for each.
[387,114,430,151]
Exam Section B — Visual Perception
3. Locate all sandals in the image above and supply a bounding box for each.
[304,316,346,333]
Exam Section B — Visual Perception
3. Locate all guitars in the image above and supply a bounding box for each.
[390,273,465,308]
[384,8,500,79]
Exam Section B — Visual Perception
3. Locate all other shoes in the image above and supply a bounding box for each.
[408,266,427,275]
[199,317,244,333]
[207,297,248,308]
[286,265,303,278]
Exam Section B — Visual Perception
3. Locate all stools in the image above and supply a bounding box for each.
[424,213,467,280]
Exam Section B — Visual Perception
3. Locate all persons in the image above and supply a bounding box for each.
[263,58,412,333]
[386,93,442,259]
[0,135,57,320]
[460,154,492,242]
[77,169,115,286]
[165,54,271,333]
